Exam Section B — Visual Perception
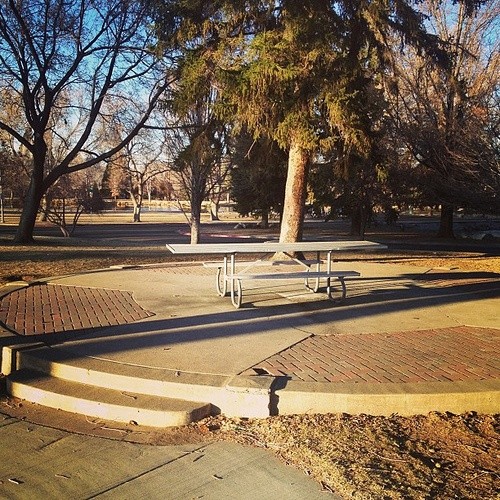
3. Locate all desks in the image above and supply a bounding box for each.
[166,239,388,301]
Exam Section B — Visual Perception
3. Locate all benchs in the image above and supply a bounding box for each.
[203,259,324,297]
[224,270,360,309]
